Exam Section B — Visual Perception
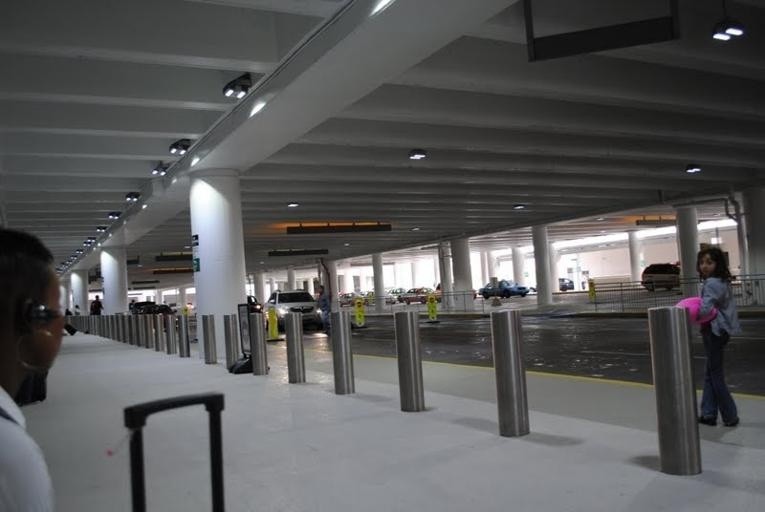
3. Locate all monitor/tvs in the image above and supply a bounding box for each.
[524,0,681,61]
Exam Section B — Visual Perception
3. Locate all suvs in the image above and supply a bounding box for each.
[259,290,324,335]
[639,262,682,294]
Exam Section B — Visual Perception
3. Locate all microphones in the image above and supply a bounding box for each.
[15,334,48,375]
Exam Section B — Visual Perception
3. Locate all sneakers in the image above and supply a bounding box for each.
[695,413,718,428]
[723,417,740,428]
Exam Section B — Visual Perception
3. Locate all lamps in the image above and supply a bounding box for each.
[409,148,425,163]
[96,226,106,234]
[151,160,170,176]
[125,192,140,202]
[108,212,121,220]
[56,237,96,271]
[223,71,252,99]
[169,138,190,156]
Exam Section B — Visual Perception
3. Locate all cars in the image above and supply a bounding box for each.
[246,295,262,315]
[128,300,194,334]
[337,280,477,309]
[530,278,586,293]
[477,279,531,301]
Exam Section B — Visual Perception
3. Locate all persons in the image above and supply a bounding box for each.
[65,309,72,315]
[317,285,331,334]
[696,246,742,427]
[0,226,65,512]
[90,295,104,315]
[75,305,81,315]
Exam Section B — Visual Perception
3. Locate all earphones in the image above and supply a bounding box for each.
[17,296,59,331]
